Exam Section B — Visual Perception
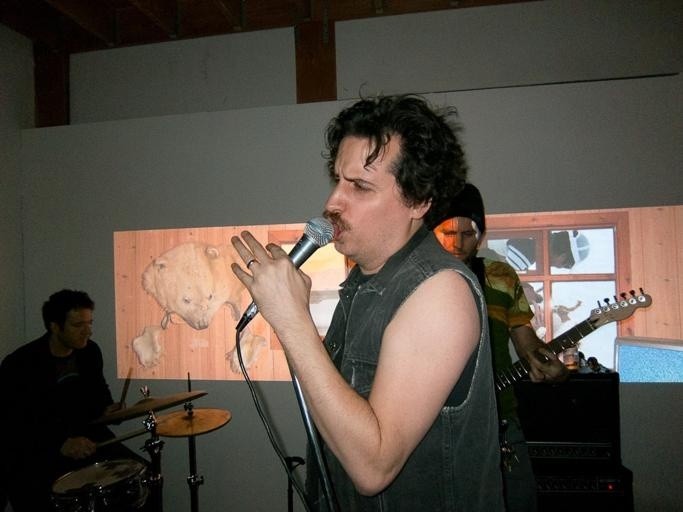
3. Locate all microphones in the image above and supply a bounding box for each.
[237,216,334,333]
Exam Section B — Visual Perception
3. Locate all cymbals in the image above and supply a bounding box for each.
[154,408,232,436]
[97,391,208,425]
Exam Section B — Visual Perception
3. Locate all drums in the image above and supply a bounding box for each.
[53,460,150,511]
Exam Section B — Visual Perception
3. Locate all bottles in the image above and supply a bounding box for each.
[563,344,579,371]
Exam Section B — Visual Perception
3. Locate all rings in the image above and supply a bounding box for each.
[544,374,545,380]
[247,260,256,268]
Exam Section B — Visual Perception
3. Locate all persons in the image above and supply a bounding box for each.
[488,229,589,330]
[232,97,506,510]
[432,184,569,512]
[0,289,127,512]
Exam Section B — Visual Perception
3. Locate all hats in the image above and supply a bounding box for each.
[568,230,592,265]
[423,182,486,235]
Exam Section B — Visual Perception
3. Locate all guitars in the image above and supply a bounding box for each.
[495,287,652,396]
